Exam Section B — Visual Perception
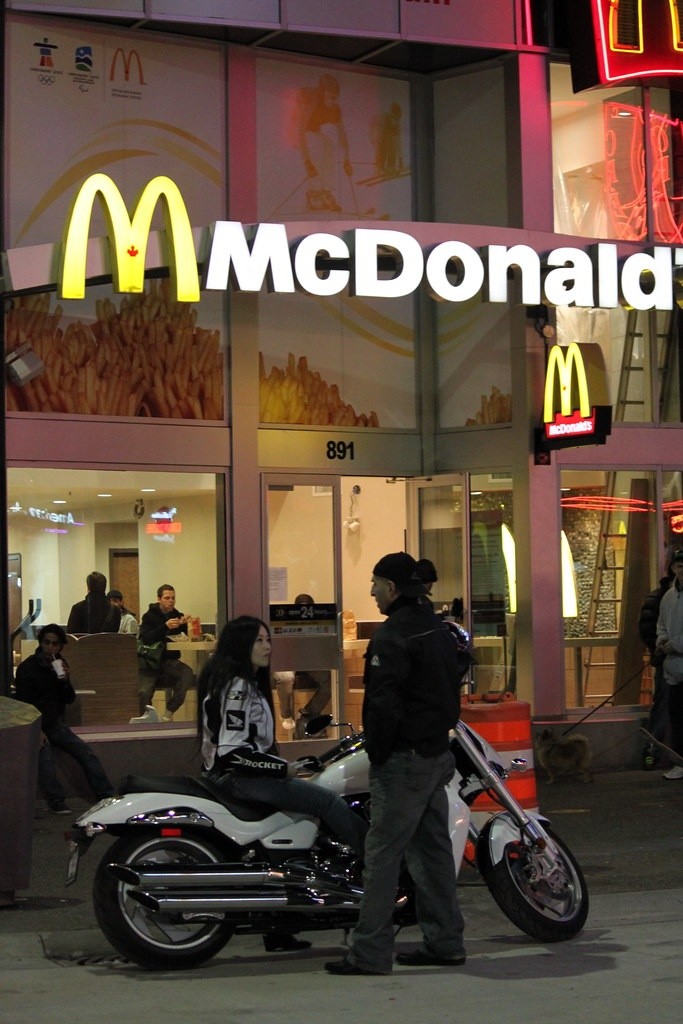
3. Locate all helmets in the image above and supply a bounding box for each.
[444,620,471,656]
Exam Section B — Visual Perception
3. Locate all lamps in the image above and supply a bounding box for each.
[534,317,554,338]
[344,516,360,530]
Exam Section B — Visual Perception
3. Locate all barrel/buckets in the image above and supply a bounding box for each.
[460,691,539,868]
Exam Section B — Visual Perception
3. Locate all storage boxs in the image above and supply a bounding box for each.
[6,342,46,388]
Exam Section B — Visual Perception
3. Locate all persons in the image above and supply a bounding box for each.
[15,624,112,815]
[272,593,331,729]
[323,551,468,974]
[66,571,195,724]
[200,614,405,951]
[637,548,683,779]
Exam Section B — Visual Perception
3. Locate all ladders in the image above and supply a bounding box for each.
[583,308,673,706]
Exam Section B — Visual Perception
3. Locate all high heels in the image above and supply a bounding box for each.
[262,933,313,952]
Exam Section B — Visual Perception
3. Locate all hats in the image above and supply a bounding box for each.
[372,552,438,584]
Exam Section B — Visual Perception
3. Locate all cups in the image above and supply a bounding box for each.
[52,659,66,679]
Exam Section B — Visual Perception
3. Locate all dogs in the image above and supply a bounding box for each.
[532,728,594,786]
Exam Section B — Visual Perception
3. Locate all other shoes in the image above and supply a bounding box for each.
[662,766,683,779]
[51,802,73,815]
[279,716,297,730]
[161,716,174,722]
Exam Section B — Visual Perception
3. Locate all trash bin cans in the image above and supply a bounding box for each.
[0,695,42,891]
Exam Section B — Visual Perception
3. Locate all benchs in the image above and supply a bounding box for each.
[154,673,200,704]
[271,673,321,740]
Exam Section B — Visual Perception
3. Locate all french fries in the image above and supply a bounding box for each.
[3,275,226,422]
[257,351,380,427]
[465,385,513,425]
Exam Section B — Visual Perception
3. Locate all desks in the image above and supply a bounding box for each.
[473,634,622,706]
[167,641,216,666]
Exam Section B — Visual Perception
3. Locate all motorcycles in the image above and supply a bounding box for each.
[69,671,589,970]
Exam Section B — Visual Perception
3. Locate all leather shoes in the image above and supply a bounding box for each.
[395,949,465,966]
[324,958,382,975]
